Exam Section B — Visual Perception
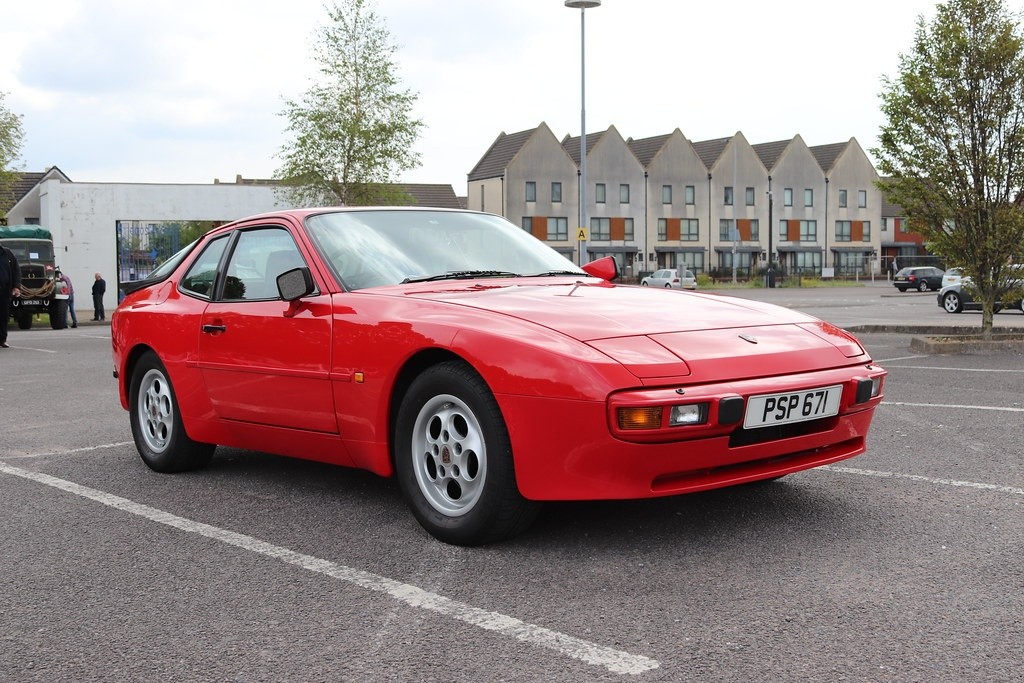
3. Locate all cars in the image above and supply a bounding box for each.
[640,267,697,291]
[937,264,1024,313]
[893,266,944,293]
[111,207,886,544]
[941,267,974,287]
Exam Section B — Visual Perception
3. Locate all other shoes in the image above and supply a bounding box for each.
[101,317,105,320]
[71,324,77,328]
[0,342,9,348]
[91,318,99,321]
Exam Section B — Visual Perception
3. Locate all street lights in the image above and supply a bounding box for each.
[767,191,776,289]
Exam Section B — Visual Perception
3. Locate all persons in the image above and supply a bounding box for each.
[0,244,22,348]
[55,270,77,329]
[91,273,106,321]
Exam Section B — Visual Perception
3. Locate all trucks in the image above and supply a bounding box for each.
[0,226,69,330]
